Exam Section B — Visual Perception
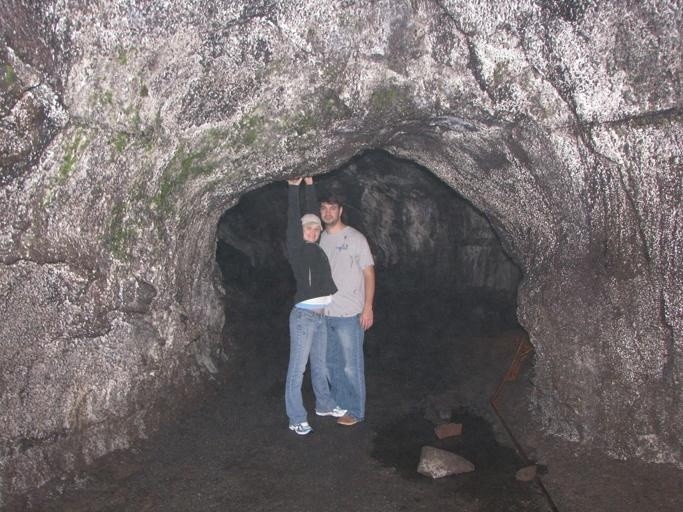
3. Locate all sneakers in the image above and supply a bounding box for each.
[288,422,314,436]
[315,405,358,426]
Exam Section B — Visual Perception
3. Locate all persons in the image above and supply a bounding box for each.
[280,176,348,436]
[318,198,375,426]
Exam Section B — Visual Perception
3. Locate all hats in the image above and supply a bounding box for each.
[300,213,323,231]
